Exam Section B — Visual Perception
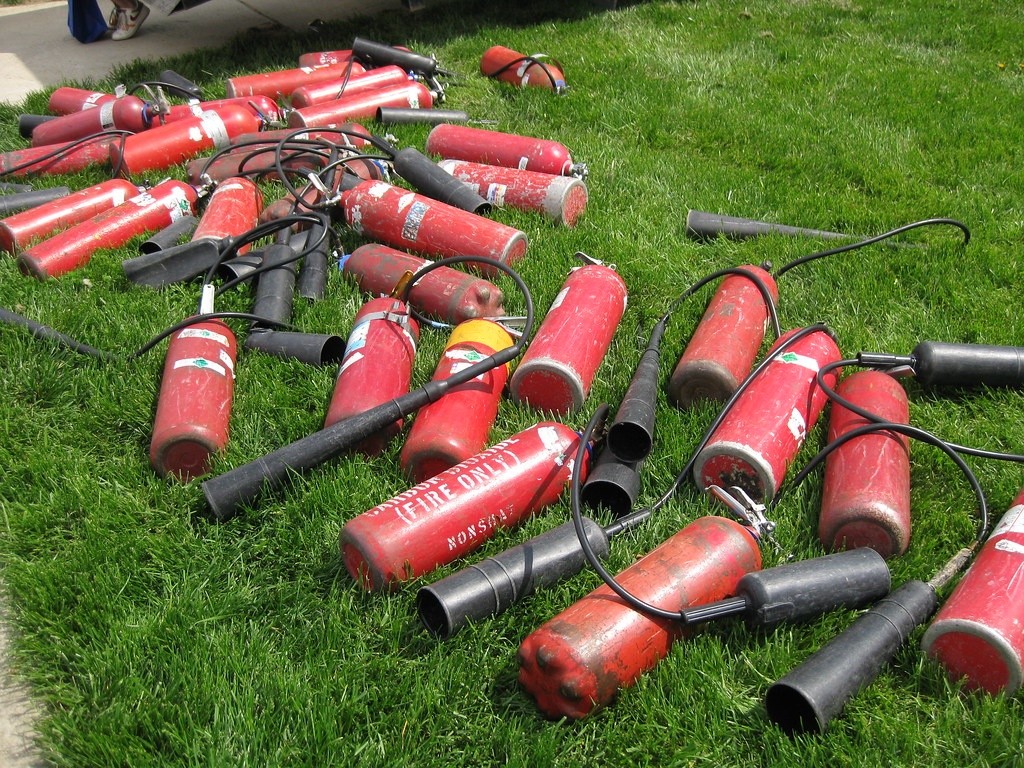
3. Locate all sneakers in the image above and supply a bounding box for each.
[107,5,122,30]
[112,1,150,40]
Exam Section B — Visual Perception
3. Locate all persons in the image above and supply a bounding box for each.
[106,0,150,40]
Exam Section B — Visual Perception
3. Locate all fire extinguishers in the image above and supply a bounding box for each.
[1,36,1024,738]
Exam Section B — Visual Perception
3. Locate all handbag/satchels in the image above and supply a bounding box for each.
[68,0,108,43]
